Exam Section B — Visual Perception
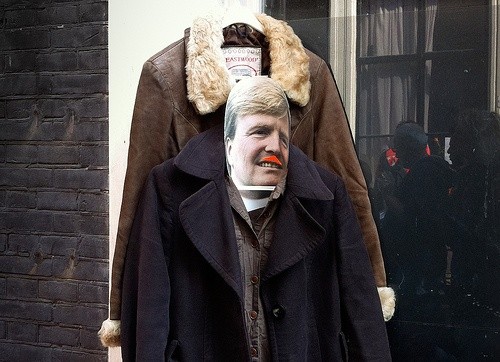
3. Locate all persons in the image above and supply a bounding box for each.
[223,77,291,187]
[358,106,500,362]
